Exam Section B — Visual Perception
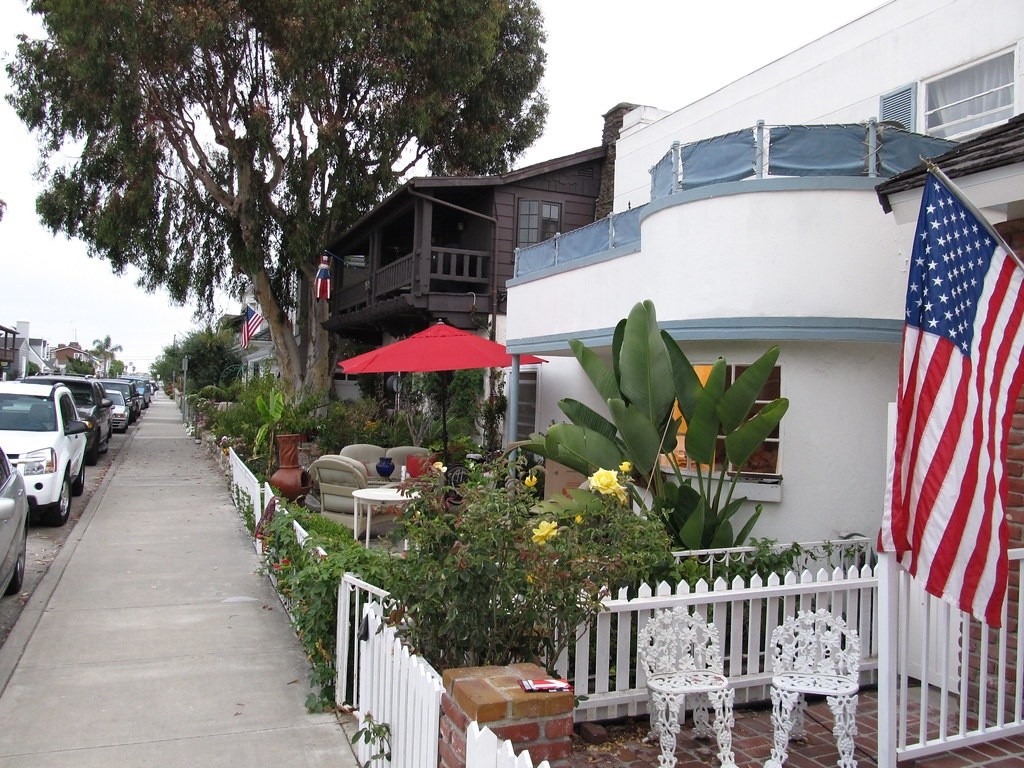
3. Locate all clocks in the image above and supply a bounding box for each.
[388,375,403,393]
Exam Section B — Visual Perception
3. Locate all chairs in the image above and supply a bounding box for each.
[763,609,861,768]
[639,608,737,768]
[29,403,50,423]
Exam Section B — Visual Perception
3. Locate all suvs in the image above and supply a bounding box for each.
[20,372,114,468]
[98,379,139,426]
[0,382,88,525]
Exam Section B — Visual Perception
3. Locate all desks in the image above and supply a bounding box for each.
[351,488,422,551]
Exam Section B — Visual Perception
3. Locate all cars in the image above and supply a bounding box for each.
[144,379,161,407]
[0,446,29,600]
[104,389,131,433]
[137,379,149,414]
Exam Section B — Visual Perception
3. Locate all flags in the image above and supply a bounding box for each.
[234,305,264,350]
[866,170,1024,631]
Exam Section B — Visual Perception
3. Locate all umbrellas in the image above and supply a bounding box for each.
[335,315,550,469]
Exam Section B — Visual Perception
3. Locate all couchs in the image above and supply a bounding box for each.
[340,445,431,490]
[314,454,371,517]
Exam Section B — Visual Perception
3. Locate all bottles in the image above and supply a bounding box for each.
[376,457,395,477]
[400,466,406,482]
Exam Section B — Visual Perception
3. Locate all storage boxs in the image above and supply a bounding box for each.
[545,456,587,503]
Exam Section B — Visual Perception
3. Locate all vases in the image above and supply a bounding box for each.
[406,456,436,478]
[270,435,312,504]
[377,456,394,482]
